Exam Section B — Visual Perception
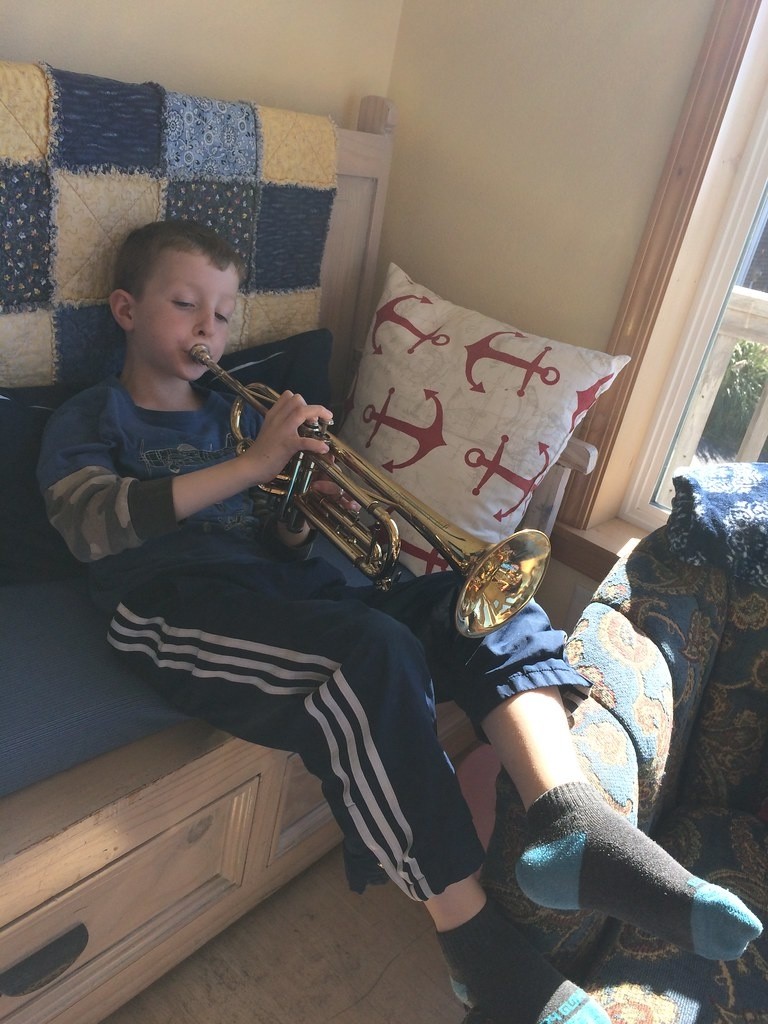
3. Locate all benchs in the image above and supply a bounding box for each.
[0,54,600,1024]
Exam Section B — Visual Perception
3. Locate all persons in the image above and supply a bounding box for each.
[36,218,762,1021]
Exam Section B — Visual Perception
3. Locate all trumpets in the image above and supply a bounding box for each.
[188,343,552,640]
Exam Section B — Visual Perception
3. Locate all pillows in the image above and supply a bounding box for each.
[334,261,634,578]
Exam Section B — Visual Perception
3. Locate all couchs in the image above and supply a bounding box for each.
[476,460,768,1024]
